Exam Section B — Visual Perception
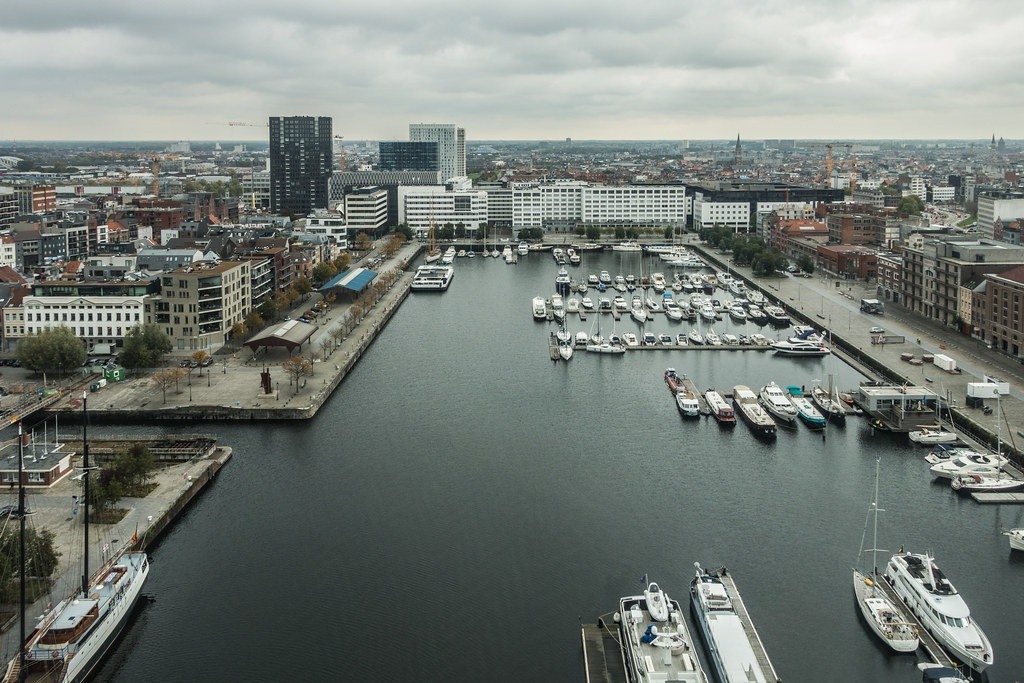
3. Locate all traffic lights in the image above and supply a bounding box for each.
[517,241,528,256]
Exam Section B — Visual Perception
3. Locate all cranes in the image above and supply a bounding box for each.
[90,152,184,197]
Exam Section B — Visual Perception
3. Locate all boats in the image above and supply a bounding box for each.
[617,580,709,683]
[796,324,821,343]
[503,245,513,264]
[772,331,832,358]
[664,367,888,434]
[1000,527,1024,550]
[930,450,1010,480]
[689,574,767,683]
[644,581,669,622]
[552,187,789,360]
[886,543,994,673]
[924,443,986,465]
[532,295,547,320]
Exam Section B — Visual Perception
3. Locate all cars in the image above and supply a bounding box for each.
[361,252,386,270]
[418,238,457,243]
[284,287,329,323]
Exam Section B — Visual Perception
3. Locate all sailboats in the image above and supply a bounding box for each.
[949,392,1024,493]
[0,390,150,683]
[424,189,500,264]
[852,456,919,653]
[810,314,847,421]
[908,371,959,444]
